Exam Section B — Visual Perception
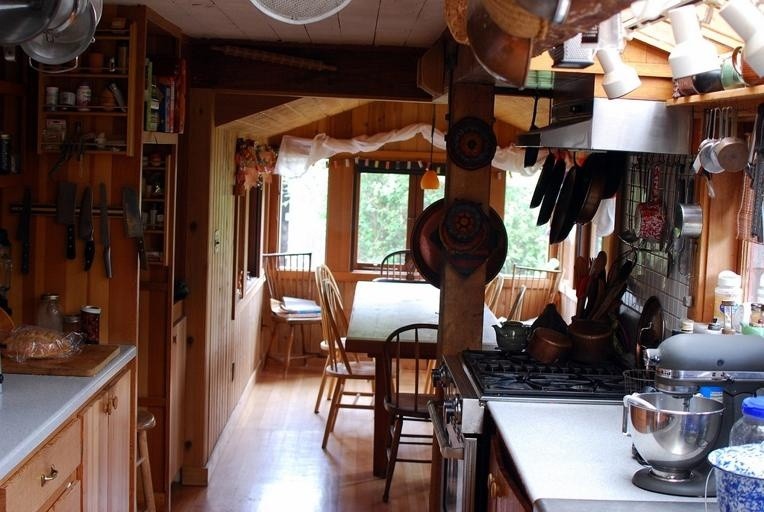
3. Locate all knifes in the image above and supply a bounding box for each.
[76,185,96,271]
[97,181,113,278]
[119,182,150,270]
[16,184,35,275]
[55,179,78,260]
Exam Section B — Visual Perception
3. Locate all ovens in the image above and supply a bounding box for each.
[426,400,480,512]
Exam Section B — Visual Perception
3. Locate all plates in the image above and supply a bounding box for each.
[446,115,499,170]
[444,198,484,245]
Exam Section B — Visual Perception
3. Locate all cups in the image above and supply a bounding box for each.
[622,368,657,435]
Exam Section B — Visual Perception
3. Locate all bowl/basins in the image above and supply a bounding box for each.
[707,442,764,511]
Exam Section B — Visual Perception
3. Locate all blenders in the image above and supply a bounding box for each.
[626,328,764,495]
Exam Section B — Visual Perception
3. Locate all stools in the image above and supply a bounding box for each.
[134,407,161,511]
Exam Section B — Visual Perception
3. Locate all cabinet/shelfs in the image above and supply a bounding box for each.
[0,416,80,512]
[484,431,533,510]
[36,11,137,156]
[76,354,137,512]
[24,129,182,487]
[140,5,186,135]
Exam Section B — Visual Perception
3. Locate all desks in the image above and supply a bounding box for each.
[344,279,502,477]
[533,495,720,511]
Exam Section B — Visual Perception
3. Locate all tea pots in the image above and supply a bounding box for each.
[493,320,529,351]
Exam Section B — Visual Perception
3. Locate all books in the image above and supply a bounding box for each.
[269,296,322,318]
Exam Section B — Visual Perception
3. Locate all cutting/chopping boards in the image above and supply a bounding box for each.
[0,342,121,378]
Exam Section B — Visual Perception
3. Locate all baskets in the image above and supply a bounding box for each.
[623,370,656,432]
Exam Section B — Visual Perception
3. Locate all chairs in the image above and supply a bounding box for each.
[321,279,400,449]
[506,283,527,322]
[313,263,375,413]
[383,323,439,503]
[259,248,341,377]
[509,263,565,323]
[380,250,424,282]
[424,275,503,394]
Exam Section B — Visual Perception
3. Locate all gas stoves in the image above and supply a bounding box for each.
[428,345,638,436]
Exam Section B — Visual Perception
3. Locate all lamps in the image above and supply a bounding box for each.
[589,0,763,100]
[418,99,442,190]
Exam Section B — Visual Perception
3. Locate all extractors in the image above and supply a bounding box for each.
[516,97,693,155]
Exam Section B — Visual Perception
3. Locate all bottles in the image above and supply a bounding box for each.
[0,132,14,174]
[729,397,764,475]
[748,302,763,328]
[152,206,165,232]
[45,81,91,112]
[64,315,81,333]
[714,270,744,324]
[80,304,100,343]
[35,293,64,332]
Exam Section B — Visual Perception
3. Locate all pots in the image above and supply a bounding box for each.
[0,0,103,75]
[523,97,541,168]
[531,146,623,244]
[674,163,704,236]
[697,105,751,177]
[633,165,666,246]
